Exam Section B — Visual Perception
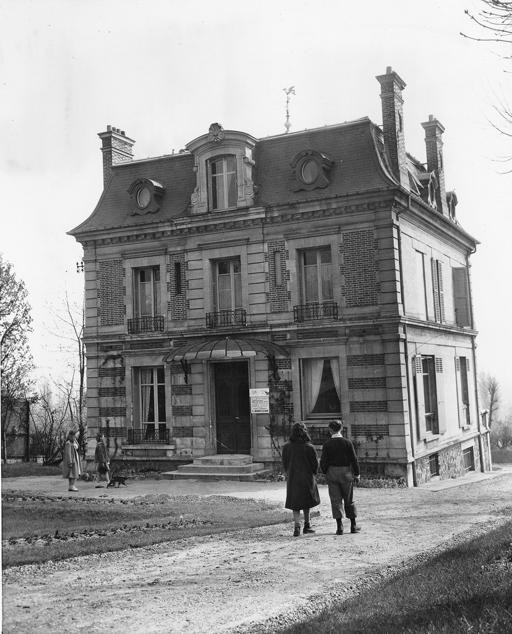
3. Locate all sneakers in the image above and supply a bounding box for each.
[68,488,79,491]
[95,485,104,488]
[336,528,361,535]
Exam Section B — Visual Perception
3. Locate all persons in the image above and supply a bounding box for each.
[318,419,363,536]
[94,430,114,489]
[279,421,319,536]
[61,429,84,492]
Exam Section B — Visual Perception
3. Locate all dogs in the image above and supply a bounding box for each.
[106,471,128,489]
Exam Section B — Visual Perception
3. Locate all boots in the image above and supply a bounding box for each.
[293,522,316,536]
